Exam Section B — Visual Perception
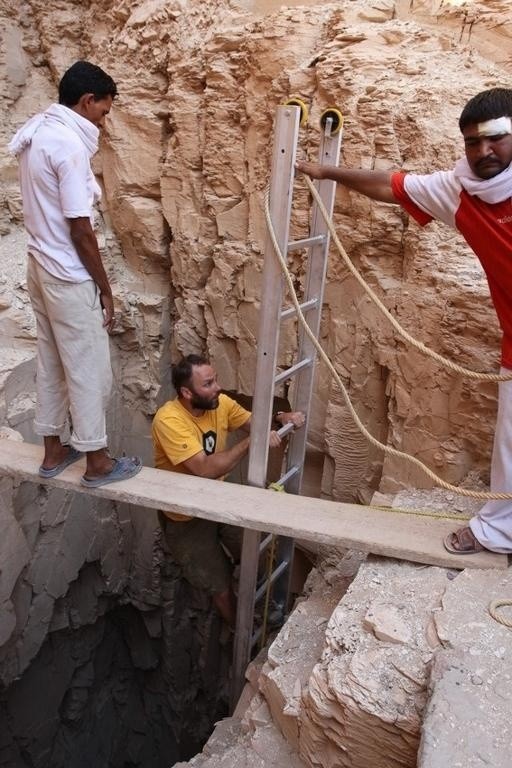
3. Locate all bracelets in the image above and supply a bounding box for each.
[272,410,286,424]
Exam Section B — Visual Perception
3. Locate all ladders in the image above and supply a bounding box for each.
[230,97,345,720]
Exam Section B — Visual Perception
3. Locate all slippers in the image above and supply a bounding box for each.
[81,454,145,489]
[443,527,486,554]
[38,443,86,478]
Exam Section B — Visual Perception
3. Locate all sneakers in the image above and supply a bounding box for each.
[257,597,286,629]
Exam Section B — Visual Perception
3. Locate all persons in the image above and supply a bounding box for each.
[149,352,308,651]
[293,85,512,558]
[5,59,145,489]
